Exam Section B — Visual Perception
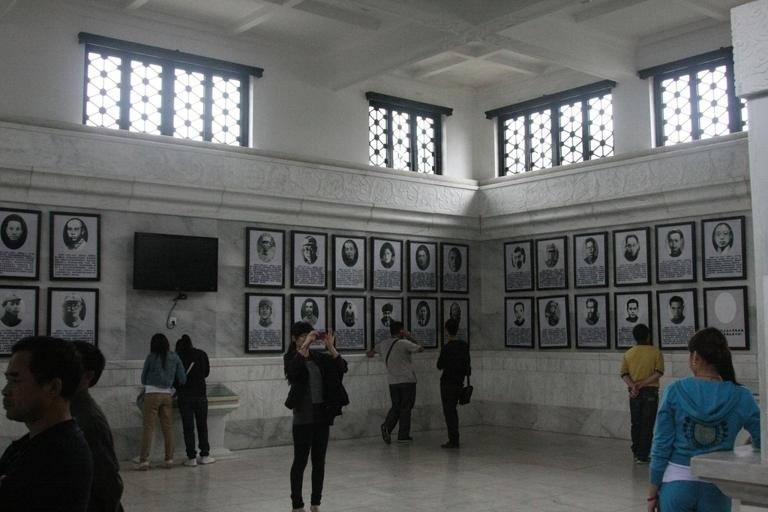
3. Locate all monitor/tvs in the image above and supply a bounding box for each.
[132,232,219,293]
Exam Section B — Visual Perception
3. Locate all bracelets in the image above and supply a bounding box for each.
[646,495,657,502]
[301,343,309,350]
[330,347,336,354]
[407,334,412,340]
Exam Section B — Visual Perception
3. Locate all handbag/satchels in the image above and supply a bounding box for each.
[137,388,145,412]
[458,385,473,405]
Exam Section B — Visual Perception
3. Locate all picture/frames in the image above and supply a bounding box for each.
[1,206,102,358]
[244,225,470,355]
[503,215,751,350]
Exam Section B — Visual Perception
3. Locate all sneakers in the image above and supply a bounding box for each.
[183,458,197,467]
[133,462,149,470]
[199,455,216,464]
[441,440,459,448]
[165,460,173,469]
[397,434,413,442]
[633,455,650,464]
[381,423,391,444]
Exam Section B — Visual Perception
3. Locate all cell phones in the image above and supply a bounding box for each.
[316,332,326,340]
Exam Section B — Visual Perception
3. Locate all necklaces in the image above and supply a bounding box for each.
[694,372,724,381]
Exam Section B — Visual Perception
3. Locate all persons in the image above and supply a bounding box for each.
[416,245,428,270]
[258,299,272,328]
[435,319,471,448]
[544,242,557,268]
[625,299,638,322]
[0,293,23,327]
[619,324,664,463]
[623,234,638,262]
[0,336,94,512]
[416,302,429,327]
[713,222,732,253]
[585,298,598,325]
[61,293,84,327]
[547,302,558,327]
[512,302,524,326]
[645,328,760,512]
[380,303,394,327]
[300,235,317,265]
[366,321,424,445]
[172,336,215,467]
[4,218,23,248]
[511,246,524,270]
[257,235,275,262]
[65,219,86,250]
[447,247,460,272]
[343,239,357,266]
[282,320,349,512]
[67,340,123,512]
[302,300,317,325]
[668,296,684,324]
[126,333,187,470]
[667,229,682,257]
[583,238,596,264]
[379,243,394,268]
[450,303,460,323]
[343,303,355,326]
